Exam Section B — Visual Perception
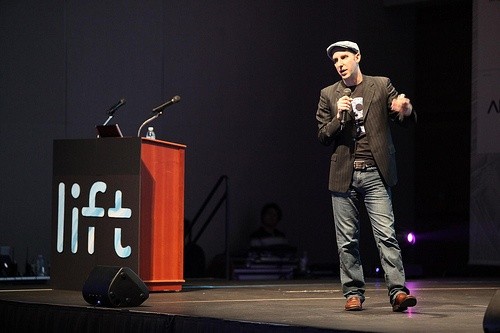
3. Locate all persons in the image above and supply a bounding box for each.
[248,202,287,253]
[316,41,418,312]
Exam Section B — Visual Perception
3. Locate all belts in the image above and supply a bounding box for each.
[354,161,368,170]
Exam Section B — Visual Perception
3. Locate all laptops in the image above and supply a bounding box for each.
[95,124,124,138]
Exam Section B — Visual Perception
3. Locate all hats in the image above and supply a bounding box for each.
[326,40,360,60]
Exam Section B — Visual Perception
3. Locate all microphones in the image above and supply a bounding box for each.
[341,88,352,126]
[153,96,181,112]
[106,98,128,115]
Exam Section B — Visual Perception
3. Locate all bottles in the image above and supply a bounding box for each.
[145,127,155,140]
[37,254,45,276]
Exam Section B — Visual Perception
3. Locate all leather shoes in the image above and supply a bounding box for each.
[393,292,417,312]
[345,297,363,311]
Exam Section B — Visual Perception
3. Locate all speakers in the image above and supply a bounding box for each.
[82,266,149,308]
[482,289,500,333]
